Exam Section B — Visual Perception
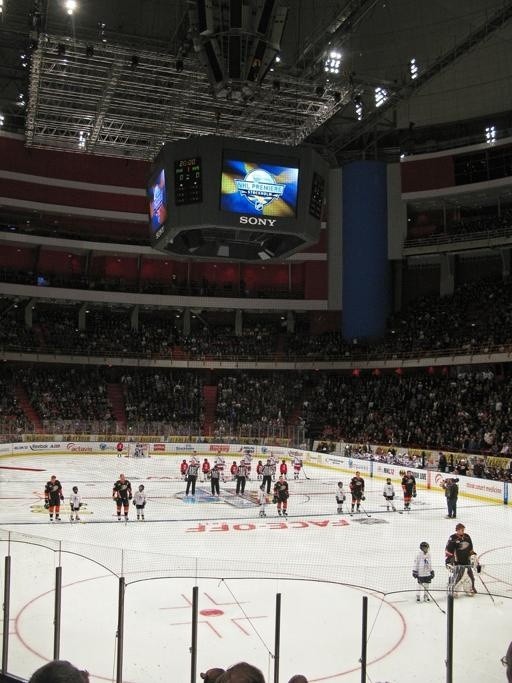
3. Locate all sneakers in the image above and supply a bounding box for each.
[425,595,430,601]
[416,594,420,602]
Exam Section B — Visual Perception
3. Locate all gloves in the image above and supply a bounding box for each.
[413,570,418,578]
[431,570,434,578]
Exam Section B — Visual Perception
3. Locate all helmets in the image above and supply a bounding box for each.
[420,542,429,554]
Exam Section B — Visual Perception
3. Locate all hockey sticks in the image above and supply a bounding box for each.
[277,498,288,522]
[417,578,446,613]
[345,501,353,516]
[472,566,504,607]
[302,467,310,480]
[388,499,403,514]
[125,503,133,526]
[199,467,204,482]
[353,493,371,517]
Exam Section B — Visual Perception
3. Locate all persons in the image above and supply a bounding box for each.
[401,470,419,510]
[334,481,347,513]
[412,541,437,602]
[348,470,367,513]
[336,274,512,489]
[65,482,85,523]
[441,206,512,240]
[180,450,312,497]
[108,473,134,522]
[255,484,270,517]
[17,658,99,683]
[1,261,345,456]
[381,477,399,512]
[502,641,512,682]
[272,475,291,516]
[197,655,312,683]
[131,480,150,521]
[39,473,67,522]
[442,521,484,598]
[441,477,461,519]
